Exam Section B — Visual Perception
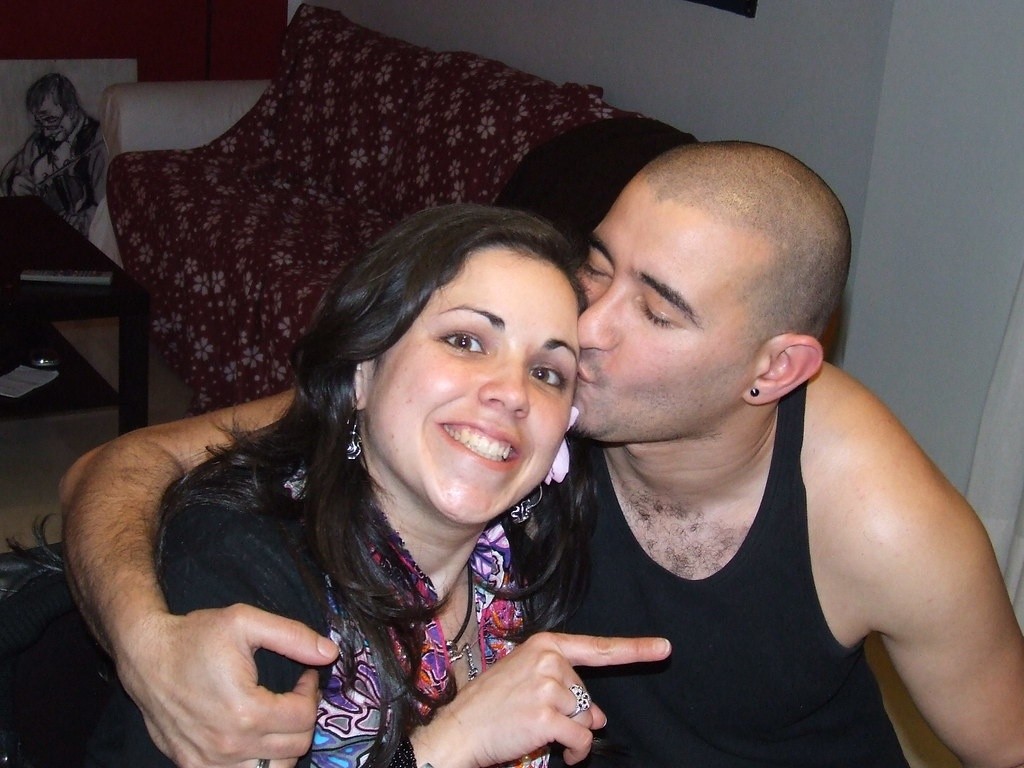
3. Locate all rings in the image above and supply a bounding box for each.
[255,759,270,768]
[565,683,591,718]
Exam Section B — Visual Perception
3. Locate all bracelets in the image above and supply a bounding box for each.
[361,737,418,768]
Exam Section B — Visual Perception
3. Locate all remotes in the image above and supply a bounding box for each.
[20,269,113,286]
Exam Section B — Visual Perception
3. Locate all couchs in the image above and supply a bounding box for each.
[86,4,841,419]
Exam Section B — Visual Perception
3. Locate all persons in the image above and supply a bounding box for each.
[56,139,1024,768]
[81,205,668,768]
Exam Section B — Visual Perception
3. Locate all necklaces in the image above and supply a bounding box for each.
[440,558,476,687]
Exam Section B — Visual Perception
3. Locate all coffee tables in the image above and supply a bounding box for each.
[0,194,152,435]
